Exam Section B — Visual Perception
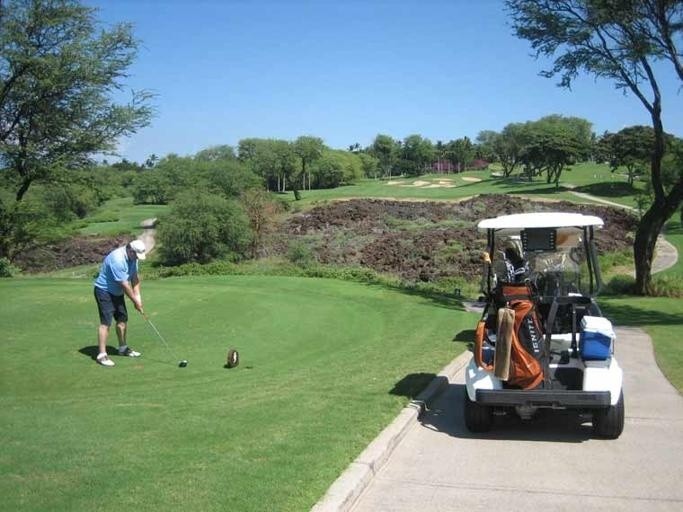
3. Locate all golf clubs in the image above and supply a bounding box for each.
[140,307,187,367]
[496,250,530,284]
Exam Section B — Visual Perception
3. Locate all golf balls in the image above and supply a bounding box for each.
[182,360,187,363]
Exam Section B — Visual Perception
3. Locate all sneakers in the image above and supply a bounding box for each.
[96,352,115,366]
[118,348,141,356]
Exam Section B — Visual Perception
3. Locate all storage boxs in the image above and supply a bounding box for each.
[577,314,617,361]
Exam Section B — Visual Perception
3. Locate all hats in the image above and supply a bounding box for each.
[129,240,146,260]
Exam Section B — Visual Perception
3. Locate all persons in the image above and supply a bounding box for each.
[91,239,146,368]
